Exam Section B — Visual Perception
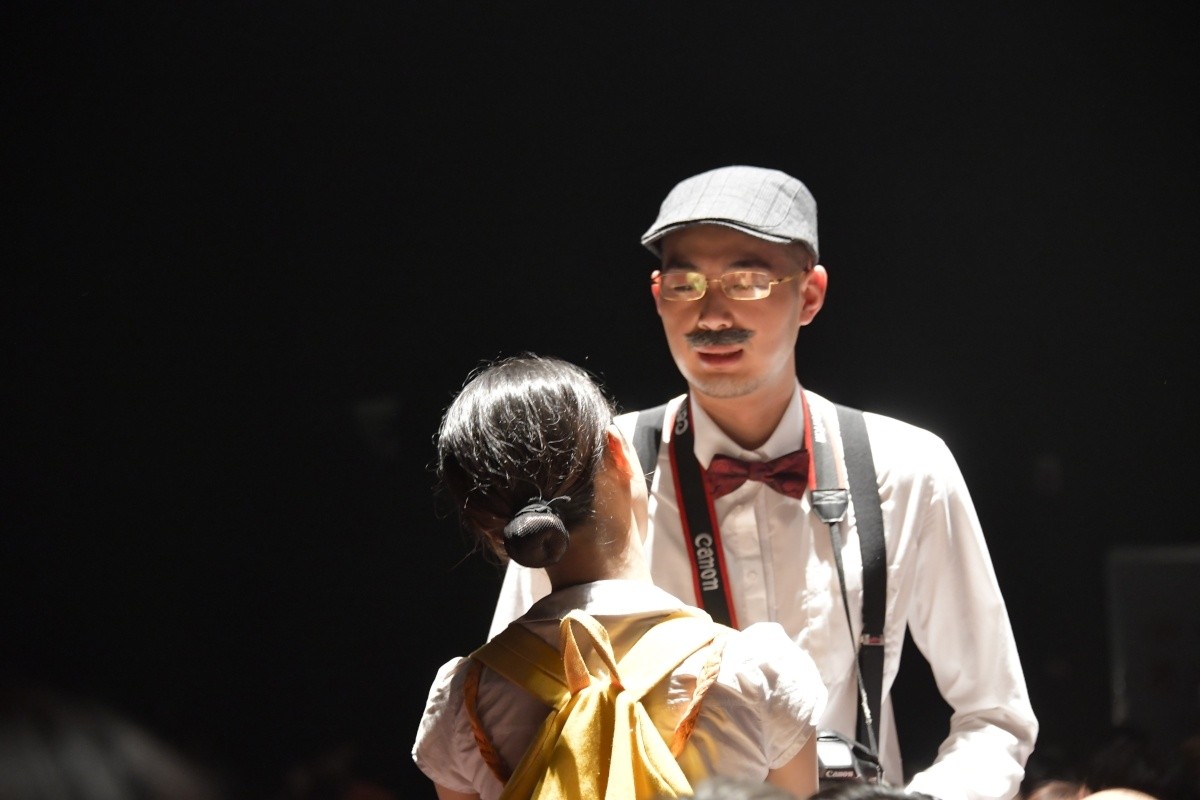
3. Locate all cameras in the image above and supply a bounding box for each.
[813,732,884,792]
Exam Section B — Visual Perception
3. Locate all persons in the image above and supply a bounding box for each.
[486,163,1040,800]
[1028,777,1158,800]
[411,349,830,800]
[806,784,939,800]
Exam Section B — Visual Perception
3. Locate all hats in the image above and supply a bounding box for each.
[642,163,821,270]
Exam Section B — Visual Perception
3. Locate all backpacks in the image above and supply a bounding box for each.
[475,608,720,800]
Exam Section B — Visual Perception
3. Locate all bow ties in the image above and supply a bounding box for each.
[707,447,813,506]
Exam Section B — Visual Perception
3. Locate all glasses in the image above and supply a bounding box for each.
[654,263,813,301]
